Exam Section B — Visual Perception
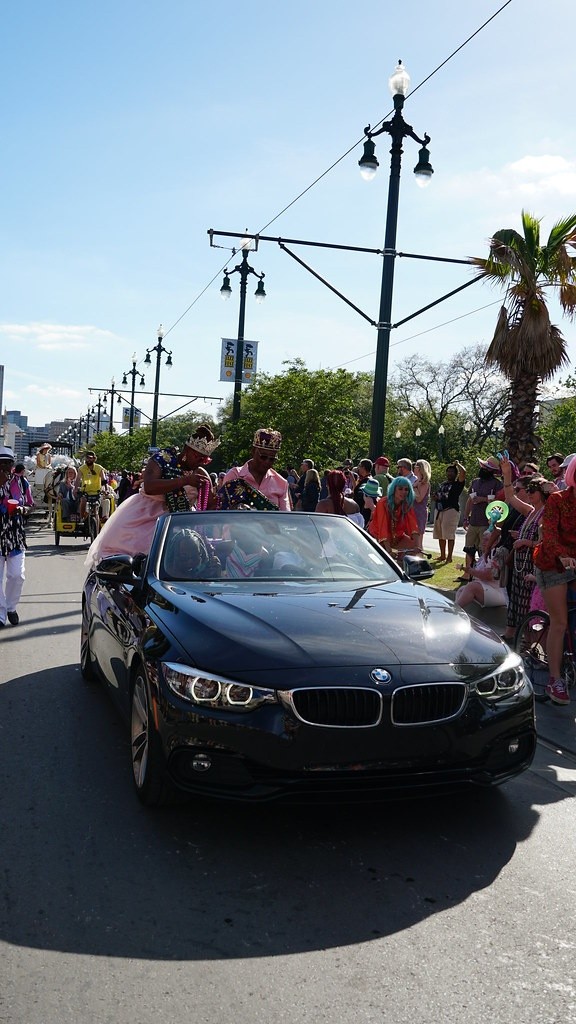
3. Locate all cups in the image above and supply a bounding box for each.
[8,499,19,514]
[487,495,495,504]
[469,492,478,504]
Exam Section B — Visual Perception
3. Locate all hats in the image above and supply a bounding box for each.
[85,451,95,458]
[252,427,284,451]
[374,457,391,467]
[477,456,503,478]
[558,453,576,467]
[361,478,382,497]
[39,443,52,452]
[0,446,14,462]
[218,472,226,478]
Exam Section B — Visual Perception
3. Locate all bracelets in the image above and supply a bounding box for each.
[464,519,467,521]
[505,484,511,487]
[532,542,533,547]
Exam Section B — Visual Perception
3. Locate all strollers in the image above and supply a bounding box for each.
[388,547,432,579]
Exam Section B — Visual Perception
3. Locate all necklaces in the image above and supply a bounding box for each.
[198,480,209,510]
[514,509,543,572]
[522,508,542,539]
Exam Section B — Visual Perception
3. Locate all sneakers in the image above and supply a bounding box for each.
[545,676,571,704]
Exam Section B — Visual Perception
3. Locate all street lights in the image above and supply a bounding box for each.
[220,230,268,424]
[415,428,422,463]
[144,325,174,444]
[439,424,445,461]
[464,420,471,451]
[360,58,433,474]
[395,430,401,462]
[57,357,122,458]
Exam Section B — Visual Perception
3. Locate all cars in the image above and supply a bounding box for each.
[77,512,538,797]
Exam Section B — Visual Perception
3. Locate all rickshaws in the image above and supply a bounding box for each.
[54,490,109,548]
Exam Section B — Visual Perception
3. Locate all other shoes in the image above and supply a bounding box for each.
[101,517,108,524]
[7,611,18,625]
[500,634,514,642]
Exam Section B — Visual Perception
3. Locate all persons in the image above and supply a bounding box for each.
[60,467,83,522]
[298,527,345,568]
[364,476,420,567]
[315,470,363,528]
[337,459,431,547]
[217,525,273,578]
[35,444,52,487]
[223,428,290,511]
[86,425,220,561]
[454,451,576,705]
[108,468,144,507]
[320,470,331,499]
[433,460,466,565]
[167,529,222,578]
[73,451,110,523]
[278,458,320,511]
[0,447,35,628]
[210,472,226,492]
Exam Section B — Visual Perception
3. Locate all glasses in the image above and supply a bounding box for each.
[526,487,540,494]
[515,486,526,493]
[521,471,533,476]
[257,448,279,463]
[397,466,403,469]
[413,465,419,469]
[196,454,206,464]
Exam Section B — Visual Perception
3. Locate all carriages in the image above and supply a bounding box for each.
[22,455,85,529]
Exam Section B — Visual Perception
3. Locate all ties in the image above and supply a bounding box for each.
[69,487,73,501]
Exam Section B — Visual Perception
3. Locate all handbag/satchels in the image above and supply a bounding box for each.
[295,498,303,511]
